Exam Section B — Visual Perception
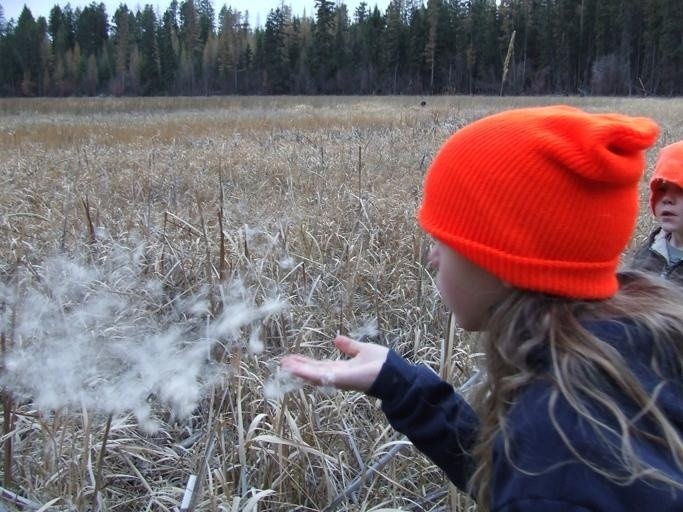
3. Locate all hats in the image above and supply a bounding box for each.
[417,104,661,299]
[649,140,683,216]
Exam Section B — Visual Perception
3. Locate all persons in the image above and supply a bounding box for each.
[630,139,683,288]
[277,104,683,512]
